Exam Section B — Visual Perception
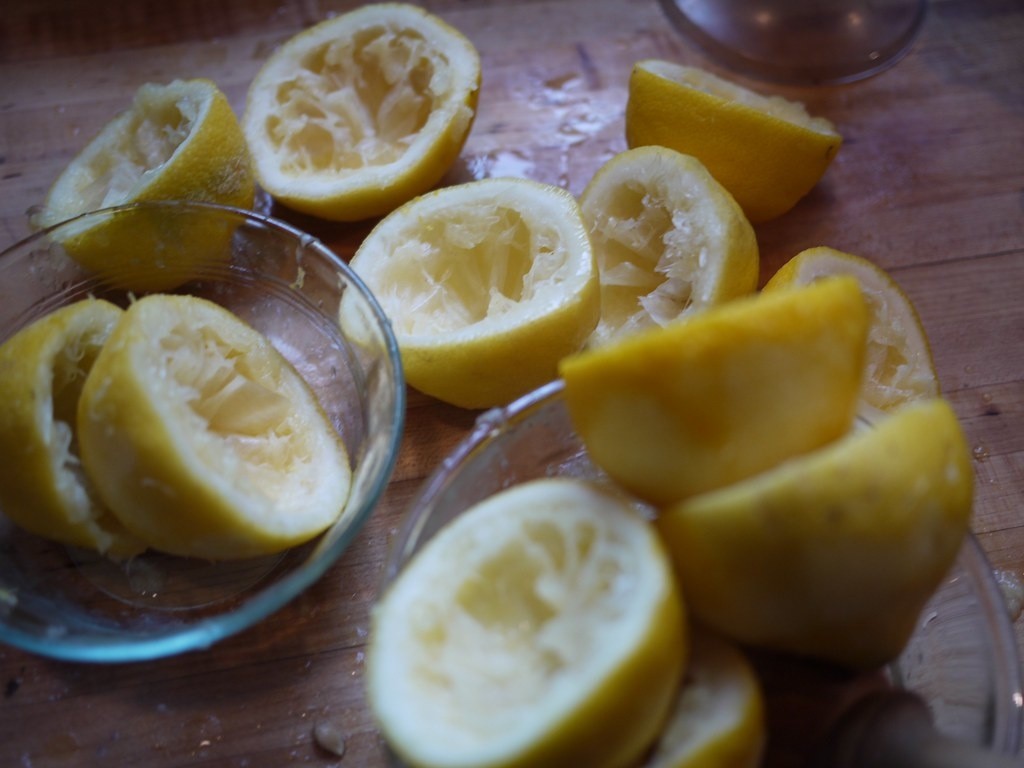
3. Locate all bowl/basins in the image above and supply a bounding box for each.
[377,371,1024,768]
[0,199,405,665]
